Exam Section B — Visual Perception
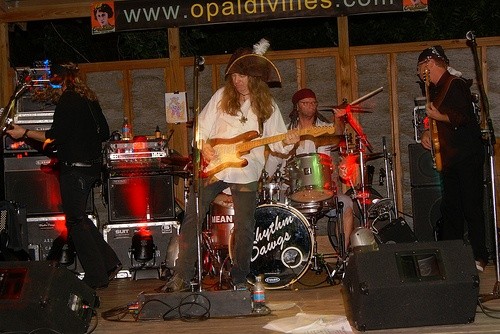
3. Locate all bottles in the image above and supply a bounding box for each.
[253,277,266,306]
[122,117,130,138]
[155,125,161,139]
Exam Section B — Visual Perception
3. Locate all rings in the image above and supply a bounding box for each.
[289,137,292,140]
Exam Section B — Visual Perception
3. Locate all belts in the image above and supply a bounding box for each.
[64,160,103,168]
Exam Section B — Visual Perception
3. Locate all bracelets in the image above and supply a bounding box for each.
[24,129,29,138]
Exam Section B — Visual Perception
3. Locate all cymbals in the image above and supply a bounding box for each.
[364,150,385,159]
[319,105,374,112]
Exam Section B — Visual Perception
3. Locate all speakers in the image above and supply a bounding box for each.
[3,156,94,215]
[0,262,96,334]
[341,240,479,330]
[107,175,175,222]
[408,144,496,252]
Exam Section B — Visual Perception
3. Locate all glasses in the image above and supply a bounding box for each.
[298,101,318,107]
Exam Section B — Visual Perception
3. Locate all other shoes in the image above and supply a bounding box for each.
[160,277,191,292]
[475,260,485,271]
[80,273,110,288]
[237,283,248,291]
[108,263,122,282]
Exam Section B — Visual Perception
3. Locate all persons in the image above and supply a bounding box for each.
[266,88,353,251]
[416,44,493,271]
[5,54,123,289]
[154,47,300,295]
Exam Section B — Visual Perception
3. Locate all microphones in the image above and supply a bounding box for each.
[195,55,205,65]
[466,31,475,41]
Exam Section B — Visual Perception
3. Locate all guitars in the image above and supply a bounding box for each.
[422,64,445,172]
[2,119,51,157]
[200,125,335,179]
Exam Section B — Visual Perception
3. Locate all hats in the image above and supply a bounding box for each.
[225,38,283,89]
[417,44,449,64]
[292,88,316,105]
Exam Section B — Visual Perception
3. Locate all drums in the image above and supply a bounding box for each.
[210,205,240,250]
[228,201,316,289]
[282,151,333,203]
[213,193,233,207]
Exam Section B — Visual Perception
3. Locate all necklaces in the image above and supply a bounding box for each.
[240,98,249,103]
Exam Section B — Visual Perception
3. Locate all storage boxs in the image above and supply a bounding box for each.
[102,220,181,279]
[15,68,58,123]
[27,215,98,280]
[413,106,427,144]
